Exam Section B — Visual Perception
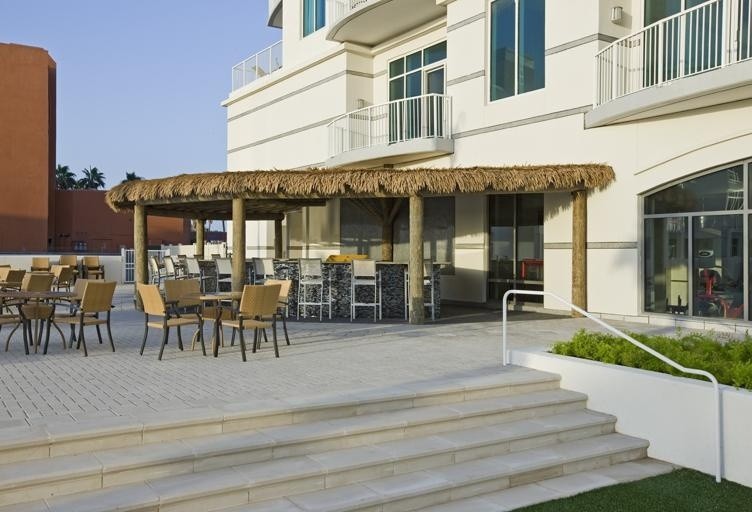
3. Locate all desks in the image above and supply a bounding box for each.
[0,292,77,353]
[179,292,243,351]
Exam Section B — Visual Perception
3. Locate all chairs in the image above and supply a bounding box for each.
[252,257,289,319]
[26,274,55,302]
[212,254,221,259]
[194,254,203,259]
[31,257,51,272]
[231,279,292,345]
[165,280,201,348]
[162,256,187,280]
[404,259,434,321]
[82,256,105,278]
[0,265,11,281]
[350,259,382,322]
[214,258,251,292]
[177,254,185,259]
[150,256,166,286]
[219,284,282,361]
[60,255,79,284]
[184,257,216,313]
[0,298,33,355]
[53,267,73,303]
[51,265,70,278]
[0,270,26,313]
[52,280,117,352]
[136,281,206,361]
[297,258,331,322]
[70,279,105,357]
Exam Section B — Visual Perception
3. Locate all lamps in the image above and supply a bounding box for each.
[612,6,624,24]
[358,99,363,107]
